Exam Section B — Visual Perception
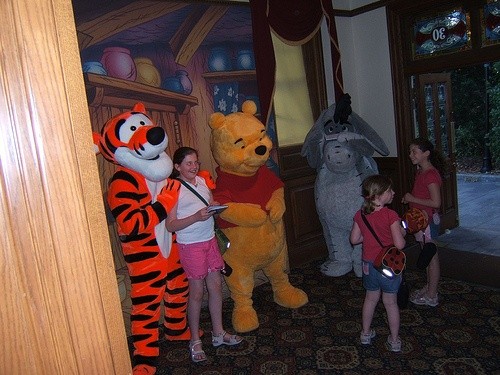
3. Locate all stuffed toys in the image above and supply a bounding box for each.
[208,100,309,333]
[301,102,389,277]
[92,103,217,375]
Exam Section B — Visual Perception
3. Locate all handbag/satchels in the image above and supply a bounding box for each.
[373,244,406,280]
[415,241,437,270]
[400,207,429,234]
[214,228,230,255]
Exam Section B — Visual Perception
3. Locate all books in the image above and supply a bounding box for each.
[207,205,228,215]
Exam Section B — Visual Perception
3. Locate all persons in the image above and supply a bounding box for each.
[350,175,407,352]
[164,147,244,362]
[401,138,445,306]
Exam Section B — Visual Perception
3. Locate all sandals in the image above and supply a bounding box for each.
[189,339,207,362]
[210,330,243,347]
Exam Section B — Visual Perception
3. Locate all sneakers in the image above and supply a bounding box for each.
[360,328,376,345]
[409,287,440,307]
[386,335,402,352]
